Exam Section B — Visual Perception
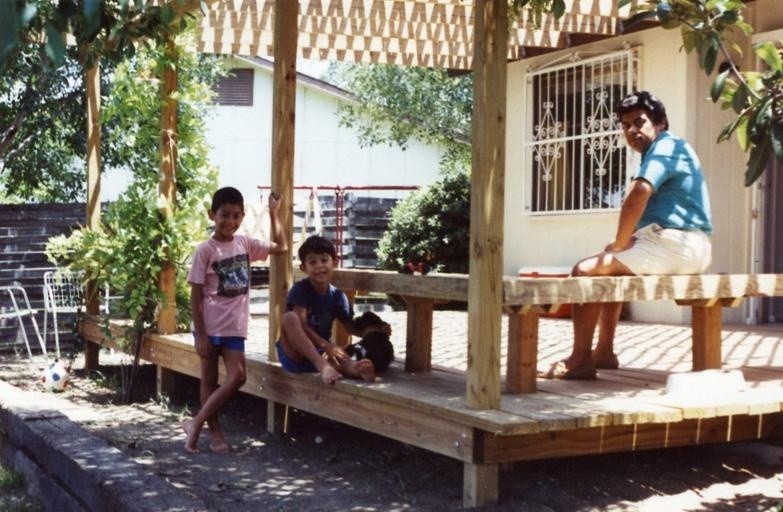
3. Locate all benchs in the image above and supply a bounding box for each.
[332,267,783,398]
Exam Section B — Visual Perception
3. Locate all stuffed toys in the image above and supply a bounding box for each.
[344,310,395,375]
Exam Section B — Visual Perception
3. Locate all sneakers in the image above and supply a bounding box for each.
[592,350,618,369]
[537,359,598,380]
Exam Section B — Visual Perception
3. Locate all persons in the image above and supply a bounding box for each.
[182,187,288,456]
[277,235,392,384]
[537,92,713,380]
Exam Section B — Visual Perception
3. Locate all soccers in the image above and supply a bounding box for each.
[41,363,70,394]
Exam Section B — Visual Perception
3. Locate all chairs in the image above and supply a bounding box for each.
[0,269,109,365]
